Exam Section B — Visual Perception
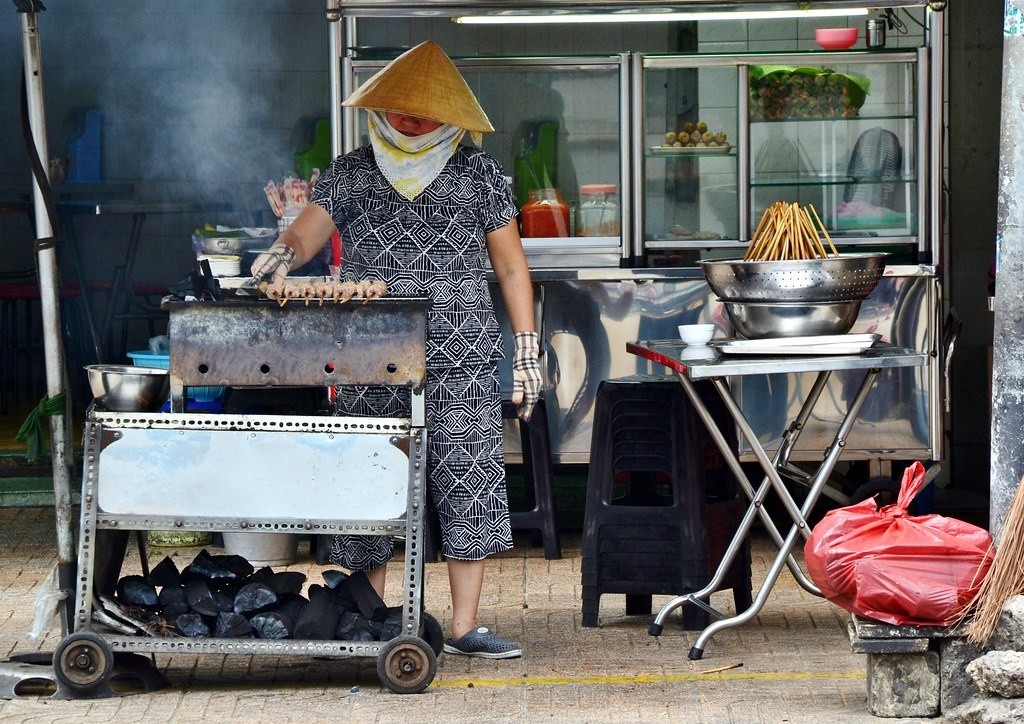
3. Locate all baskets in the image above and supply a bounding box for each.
[127,349,226,402]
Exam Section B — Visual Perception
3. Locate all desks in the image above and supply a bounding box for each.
[626,335,930,657]
[0,197,238,374]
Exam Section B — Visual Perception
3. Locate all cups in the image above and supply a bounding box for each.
[866,19,886,48]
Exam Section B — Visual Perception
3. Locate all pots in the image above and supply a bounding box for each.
[192,235,279,257]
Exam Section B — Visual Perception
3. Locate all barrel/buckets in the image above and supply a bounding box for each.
[146,531,215,547]
[222,532,298,567]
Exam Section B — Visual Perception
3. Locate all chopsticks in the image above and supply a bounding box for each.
[263,168,320,218]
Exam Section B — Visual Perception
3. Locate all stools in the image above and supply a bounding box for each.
[580,378,751,626]
[495,401,564,561]
[0,277,174,397]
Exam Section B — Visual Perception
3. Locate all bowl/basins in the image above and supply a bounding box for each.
[680,345,718,360]
[815,28,858,50]
[716,297,871,340]
[677,324,715,346]
[82,363,170,413]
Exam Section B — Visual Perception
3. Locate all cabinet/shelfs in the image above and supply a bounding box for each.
[327,0,945,464]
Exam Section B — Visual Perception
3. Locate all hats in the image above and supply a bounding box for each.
[340,41,494,132]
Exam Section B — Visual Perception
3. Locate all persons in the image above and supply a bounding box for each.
[249,40,542,659]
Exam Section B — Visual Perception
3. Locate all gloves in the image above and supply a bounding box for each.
[249,243,298,287]
[512,332,543,422]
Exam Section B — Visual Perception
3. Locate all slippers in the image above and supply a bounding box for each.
[444,626,523,658]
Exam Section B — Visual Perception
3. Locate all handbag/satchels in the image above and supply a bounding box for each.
[805,462,994,625]
